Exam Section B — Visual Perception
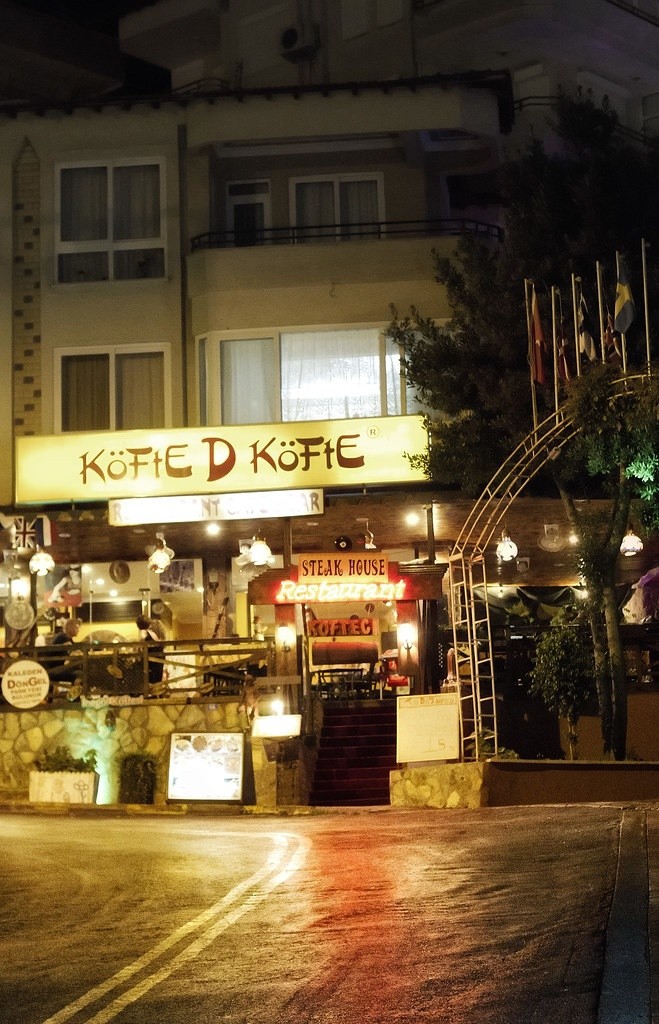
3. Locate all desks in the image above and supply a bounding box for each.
[317,669,369,701]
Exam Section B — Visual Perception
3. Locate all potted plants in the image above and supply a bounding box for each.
[28,746,100,804]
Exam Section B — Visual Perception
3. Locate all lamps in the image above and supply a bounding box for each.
[397,617,417,649]
[363,521,377,549]
[620,522,643,556]
[277,620,292,653]
[147,532,175,573]
[29,543,55,577]
[235,527,275,581]
[249,698,301,740]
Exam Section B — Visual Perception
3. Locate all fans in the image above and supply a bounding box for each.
[537,523,567,552]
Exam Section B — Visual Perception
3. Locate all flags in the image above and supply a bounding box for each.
[552,288,573,383]
[576,276,600,366]
[601,283,623,369]
[528,287,554,387]
[610,261,638,334]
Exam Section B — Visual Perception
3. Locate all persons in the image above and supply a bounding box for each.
[41,617,83,704]
[135,615,167,684]
